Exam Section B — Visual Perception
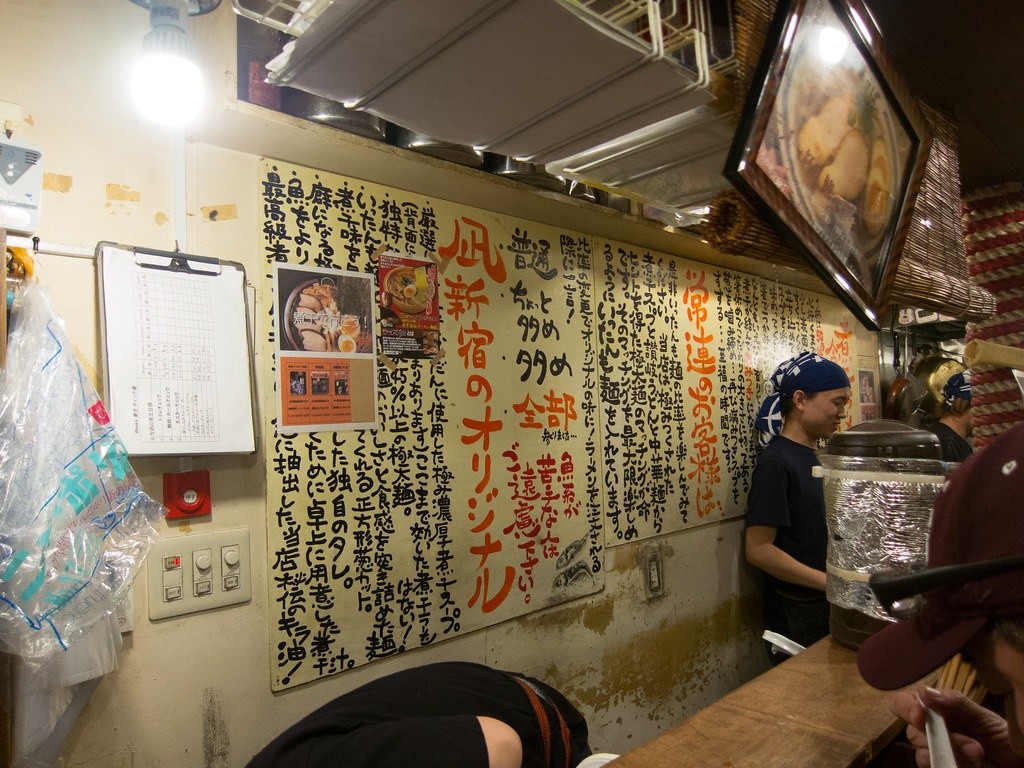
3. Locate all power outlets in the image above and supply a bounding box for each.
[648,553,663,599]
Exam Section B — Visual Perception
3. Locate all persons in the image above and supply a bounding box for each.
[929,363,976,464]
[742,351,852,667]
[853,418,1024,767]
[244,660,595,768]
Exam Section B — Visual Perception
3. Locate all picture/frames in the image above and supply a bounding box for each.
[722,0,937,332]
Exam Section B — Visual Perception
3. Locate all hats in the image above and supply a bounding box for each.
[855,420,1023,692]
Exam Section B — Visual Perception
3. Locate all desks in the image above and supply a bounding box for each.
[599,638,946,768]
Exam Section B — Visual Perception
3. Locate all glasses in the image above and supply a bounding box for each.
[870,555,1023,619]
